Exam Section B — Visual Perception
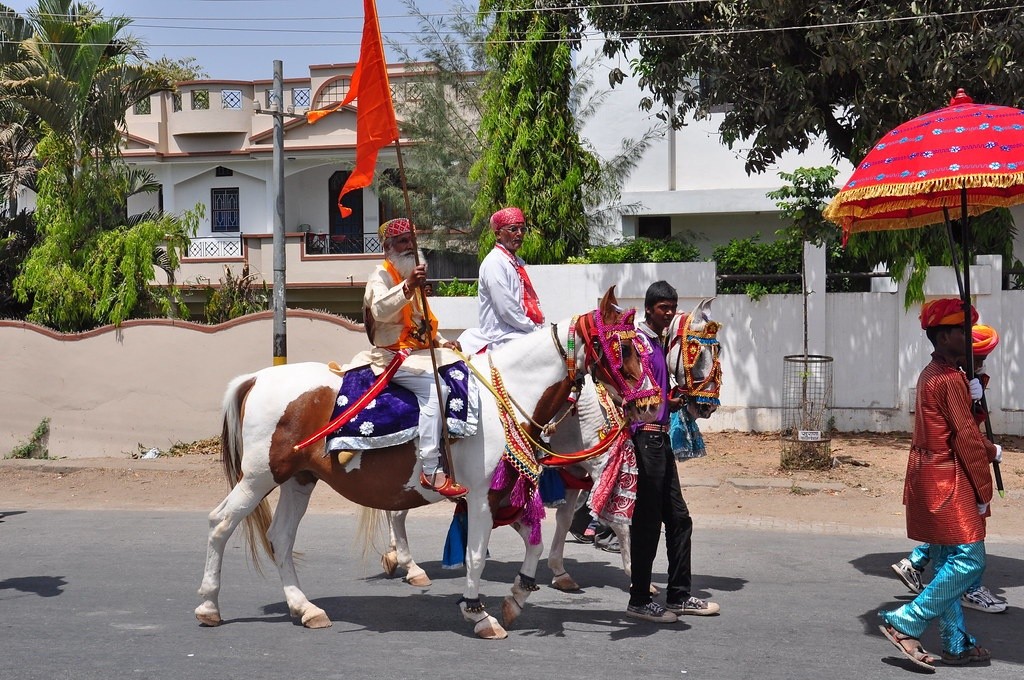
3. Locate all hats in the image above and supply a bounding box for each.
[972,324,999,356]
[918,298,979,330]
[490,208,526,234]
[378,218,416,247]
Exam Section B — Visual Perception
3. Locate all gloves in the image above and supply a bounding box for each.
[969,378,983,400]
[993,443,1003,463]
[977,502,989,515]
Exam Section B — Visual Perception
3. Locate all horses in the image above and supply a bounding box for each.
[193,284,661,639]
[504,299,722,622]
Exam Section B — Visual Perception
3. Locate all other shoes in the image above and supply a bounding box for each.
[595,537,621,553]
[570,527,594,543]
[419,471,469,498]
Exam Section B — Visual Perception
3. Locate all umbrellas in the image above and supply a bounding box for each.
[837,204,1004,495]
[823,88,1024,425]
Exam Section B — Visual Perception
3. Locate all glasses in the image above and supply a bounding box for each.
[397,237,417,244]
[501,227,527,234]
[425,289,433,292]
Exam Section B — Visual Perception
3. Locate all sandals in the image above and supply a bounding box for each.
[941,645,992,664]
[878,619,935,671]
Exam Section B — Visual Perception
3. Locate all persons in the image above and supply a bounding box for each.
[891,324,1007,614]
[365,218,468,498]
[479,206,546,348]
[624,280,720,624]
[878,299,993,672]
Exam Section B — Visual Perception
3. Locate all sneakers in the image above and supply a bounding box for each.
[664,596,720,616]
[959,585,1006,613]
[891,558,924,594]
[625,602,677,623]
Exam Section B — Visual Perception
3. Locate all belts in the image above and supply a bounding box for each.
[640,423,670,433]
